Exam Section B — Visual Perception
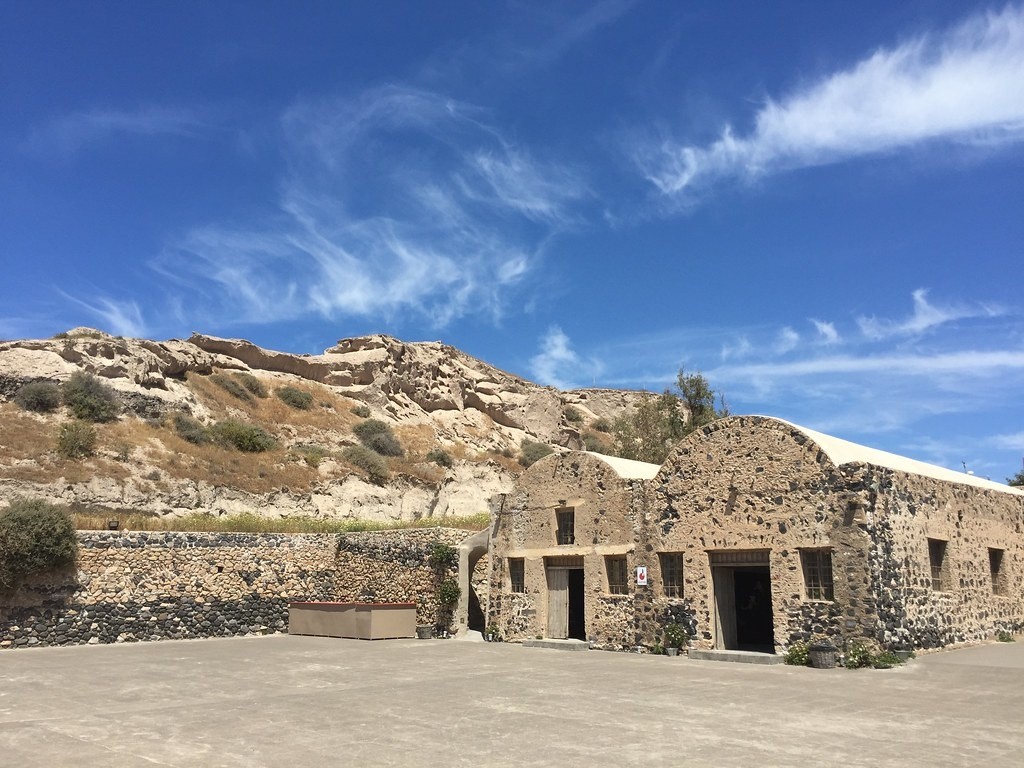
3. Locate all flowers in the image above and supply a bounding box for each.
[664,623,685,648]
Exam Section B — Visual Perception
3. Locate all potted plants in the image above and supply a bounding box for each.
[485,625,500,640]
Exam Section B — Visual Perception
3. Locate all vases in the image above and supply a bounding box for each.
[667,648,678,656]
[893,650,909,660]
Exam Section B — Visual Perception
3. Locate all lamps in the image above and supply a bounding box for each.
[109,521,119,530]
